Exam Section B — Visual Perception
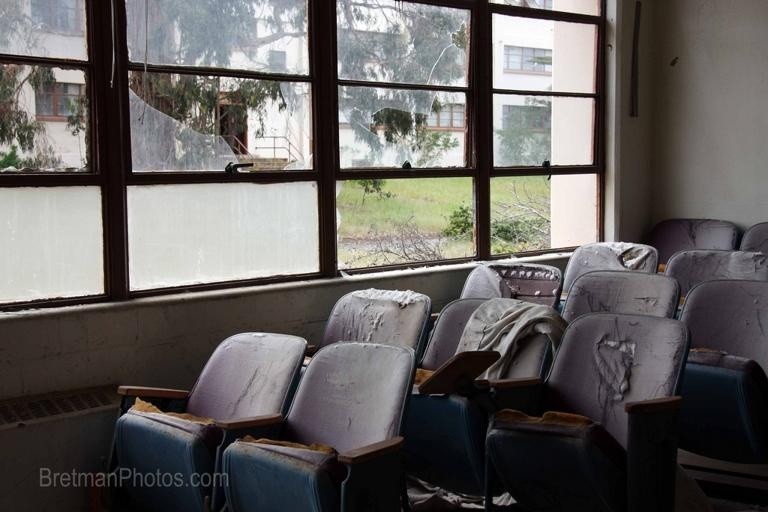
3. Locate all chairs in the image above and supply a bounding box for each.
[101,217,768,512]
